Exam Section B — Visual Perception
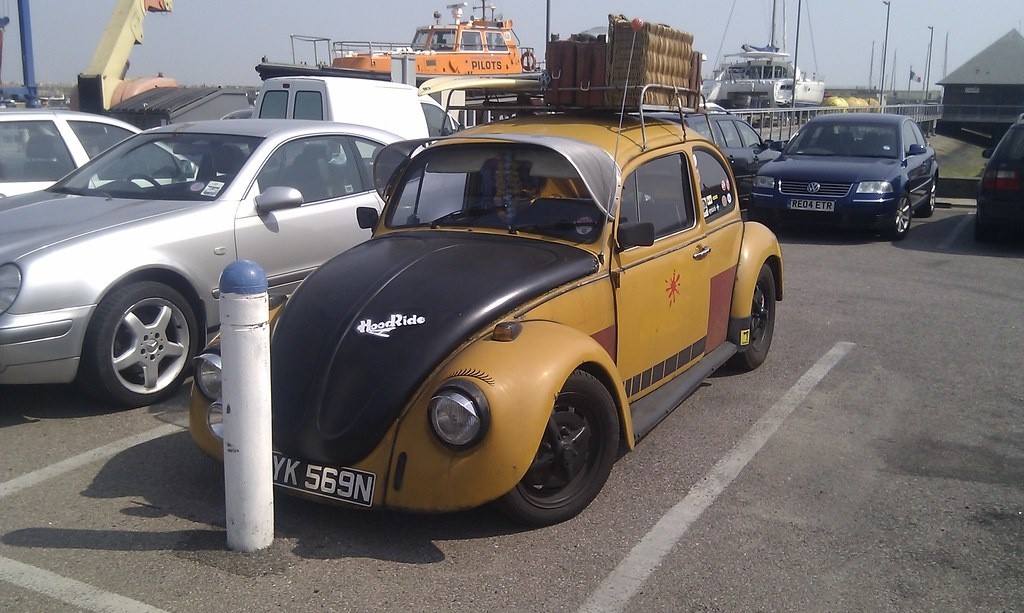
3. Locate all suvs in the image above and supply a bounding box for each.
[622,111,782,207]
[976,113,1024,241]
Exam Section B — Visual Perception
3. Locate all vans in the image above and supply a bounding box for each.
[252,76,461,160]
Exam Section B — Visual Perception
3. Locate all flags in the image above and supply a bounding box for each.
[910,72,921,82]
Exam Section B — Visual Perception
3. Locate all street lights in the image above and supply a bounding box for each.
[879,0,891,106]
[925,25,934,104]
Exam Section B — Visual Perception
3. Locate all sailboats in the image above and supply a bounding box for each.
[700,0,825,111]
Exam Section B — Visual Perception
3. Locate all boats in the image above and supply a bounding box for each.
[253,1,546,104]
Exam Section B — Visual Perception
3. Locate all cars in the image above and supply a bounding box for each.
[1,108,221,200]
[0,117,468,408]
[187,112,783,531]
[747,112,939,241]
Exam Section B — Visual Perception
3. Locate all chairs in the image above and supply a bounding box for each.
[23,134,60,177]
[291,152,333,195]
[478,160,533,215]
[200,146,244,188]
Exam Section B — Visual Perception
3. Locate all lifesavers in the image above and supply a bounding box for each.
[521,52,536,71]
